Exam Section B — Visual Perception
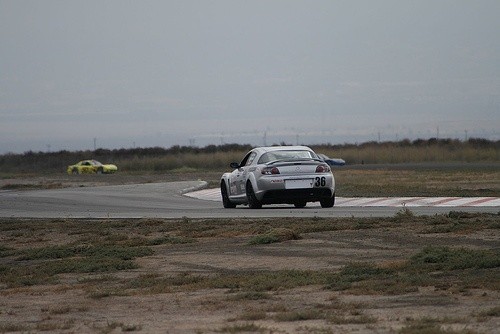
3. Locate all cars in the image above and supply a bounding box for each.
[318,153,346,166]
[66,159,118,175]
[218,145,337,209]
